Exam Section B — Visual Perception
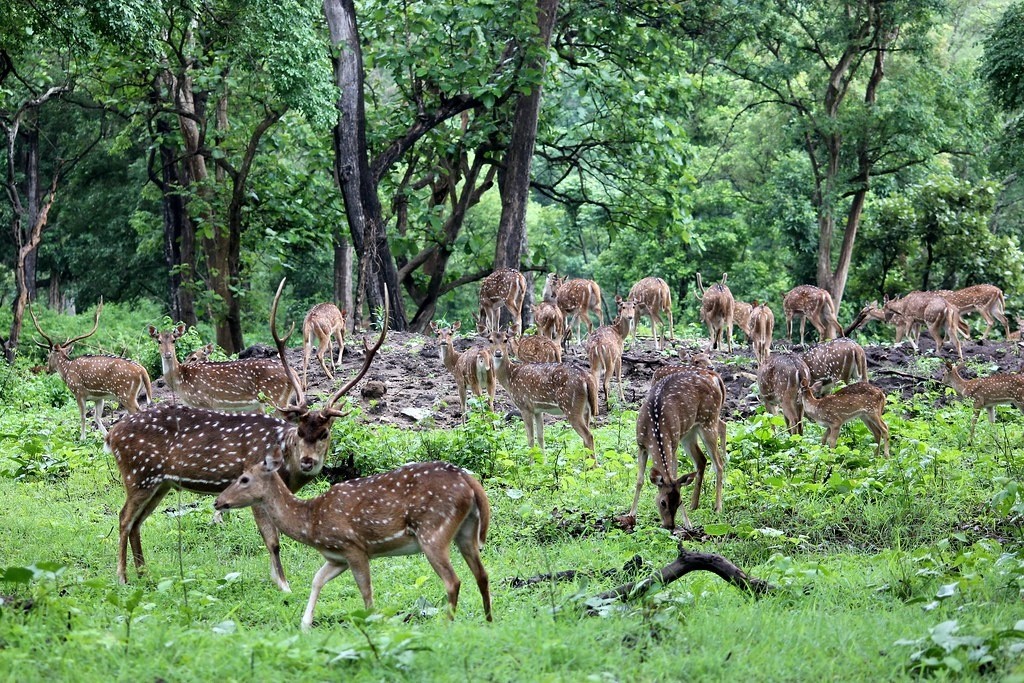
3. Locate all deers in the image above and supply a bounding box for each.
[25,266,1023,633]
